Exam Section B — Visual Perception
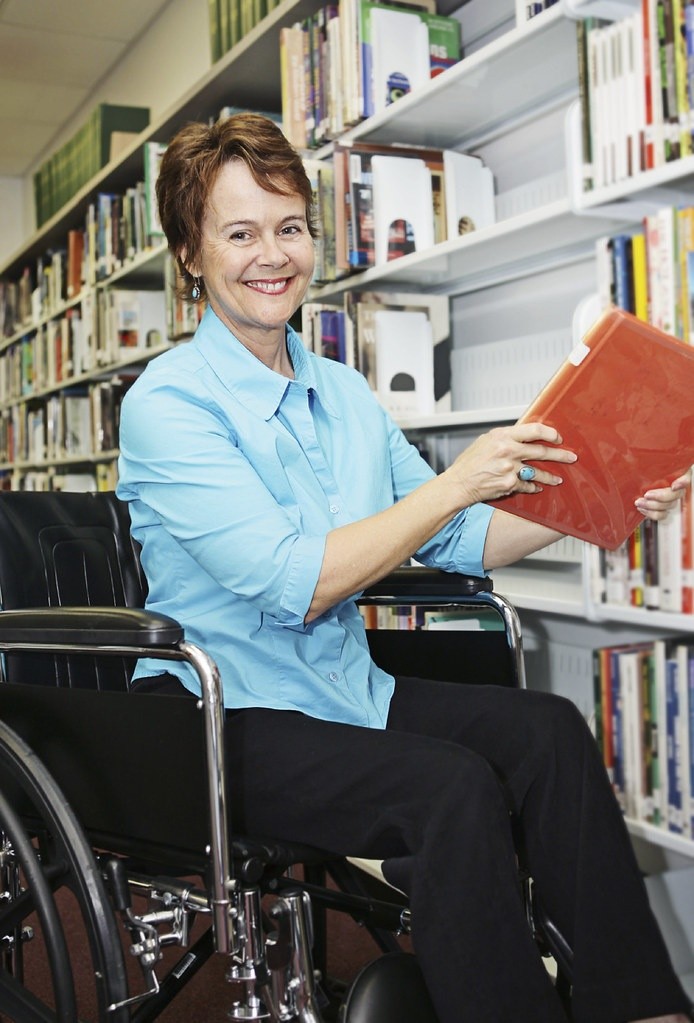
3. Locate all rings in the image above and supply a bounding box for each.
[517,465,535,481]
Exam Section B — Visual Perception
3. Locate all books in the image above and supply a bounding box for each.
[277,0,494,474]
[575,0,694,194]
[359,593,505,631]
[478,303,694,551]
[208,0,280,66]
[595,202,694,348]
[579,462,694,841]
[0,103,210,493]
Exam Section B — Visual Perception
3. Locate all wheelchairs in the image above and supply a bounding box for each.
[0,487,533,1023]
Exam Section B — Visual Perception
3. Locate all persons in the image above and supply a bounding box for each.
[115,112,694,1023]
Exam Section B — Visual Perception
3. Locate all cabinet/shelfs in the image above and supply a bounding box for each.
[0,0,694,860]
